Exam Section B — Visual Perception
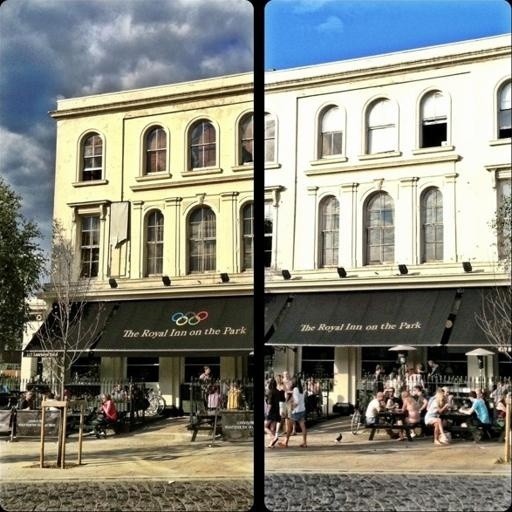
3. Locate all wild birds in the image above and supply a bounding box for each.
[336,432,343,442]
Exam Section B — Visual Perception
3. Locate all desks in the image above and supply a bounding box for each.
[191,411,222,440]
[365,409,500,443]
[68,410,117,438]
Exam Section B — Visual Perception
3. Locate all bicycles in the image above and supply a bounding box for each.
[144,387,166,415]
[350,392,373,434]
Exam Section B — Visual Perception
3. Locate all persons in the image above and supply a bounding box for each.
[93,393,118,438]
[199,365,321,448]
[19,390,34,410]
[46,392,59,434]
[364,359,507,446]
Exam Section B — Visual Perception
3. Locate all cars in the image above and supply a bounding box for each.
[1,384,22,407]
